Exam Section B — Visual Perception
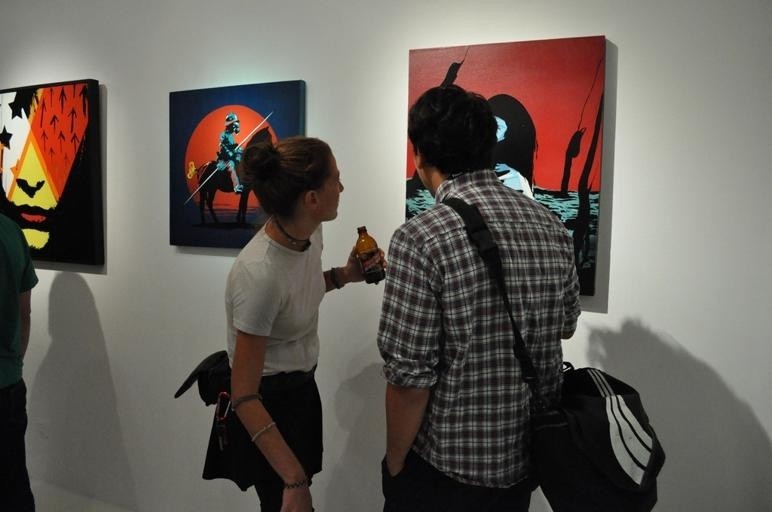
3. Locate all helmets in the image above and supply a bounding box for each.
[224,112,239,126]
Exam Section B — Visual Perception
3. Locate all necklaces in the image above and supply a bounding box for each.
[271,212,310,248]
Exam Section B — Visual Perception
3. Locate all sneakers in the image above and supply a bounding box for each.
[234,184,243,193]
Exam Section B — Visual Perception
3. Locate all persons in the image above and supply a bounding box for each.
[1,211,39,512]
[227,136,387,511]
[378,83,583,509]
[215,111,245,194]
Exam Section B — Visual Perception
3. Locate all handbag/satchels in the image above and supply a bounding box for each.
[522,366,666,512]
[201,389,256,494]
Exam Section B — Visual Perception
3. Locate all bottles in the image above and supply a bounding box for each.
[355,225,386,284]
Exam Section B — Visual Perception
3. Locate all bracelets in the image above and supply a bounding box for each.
[328,267,343,290]
[229,391,262,409]
[280,477,308,488]
[249,420,276,442]
